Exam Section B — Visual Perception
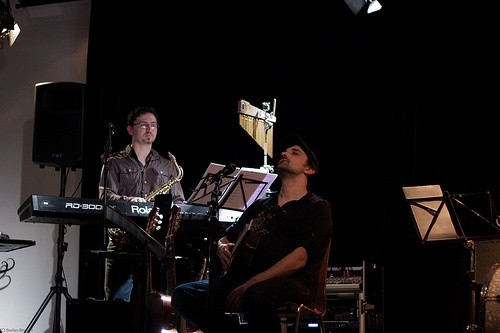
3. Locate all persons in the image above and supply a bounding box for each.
[98,108,185,301]
[171,139,332,333]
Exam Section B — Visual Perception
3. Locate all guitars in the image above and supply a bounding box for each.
[224,210,272,276]
[165,204,186,333]
[145,206,177,333]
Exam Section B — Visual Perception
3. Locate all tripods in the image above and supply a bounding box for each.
[24,167,74,333]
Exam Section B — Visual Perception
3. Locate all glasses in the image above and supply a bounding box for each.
[132,123,159,129]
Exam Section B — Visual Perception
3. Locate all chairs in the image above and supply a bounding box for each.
[277,237,332,333]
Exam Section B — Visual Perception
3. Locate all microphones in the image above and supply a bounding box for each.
[200,163,236,189]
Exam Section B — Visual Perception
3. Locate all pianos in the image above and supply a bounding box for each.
[17,194,209,298]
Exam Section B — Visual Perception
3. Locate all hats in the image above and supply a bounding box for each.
[286,134,318,172]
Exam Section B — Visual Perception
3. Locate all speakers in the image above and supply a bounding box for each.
[66,298,140,333]
[32,82,84,171]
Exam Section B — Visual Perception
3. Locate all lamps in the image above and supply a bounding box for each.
[0,0,21,47]
